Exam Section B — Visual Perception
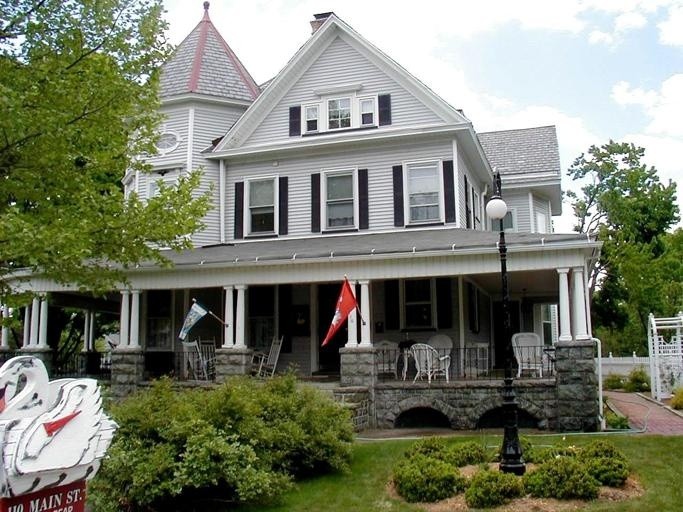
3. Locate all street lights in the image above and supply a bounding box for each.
[485,166,526,475]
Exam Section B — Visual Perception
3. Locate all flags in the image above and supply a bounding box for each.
[321,278,358,349]
[177,297,210,342]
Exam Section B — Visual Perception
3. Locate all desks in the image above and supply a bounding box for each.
[398,347,414,381]
[464,342,489,378]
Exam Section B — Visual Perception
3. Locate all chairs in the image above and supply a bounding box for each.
[511,332,544,379]
[427,334,453,369]
[410,344,452,385]
[181,335,216,381]
[108,341,117,350]
[374,340,401,381]
[249,335,284,380]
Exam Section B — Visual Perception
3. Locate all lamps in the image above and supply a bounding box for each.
[198,238,545,267]
[135,263,141,269]
